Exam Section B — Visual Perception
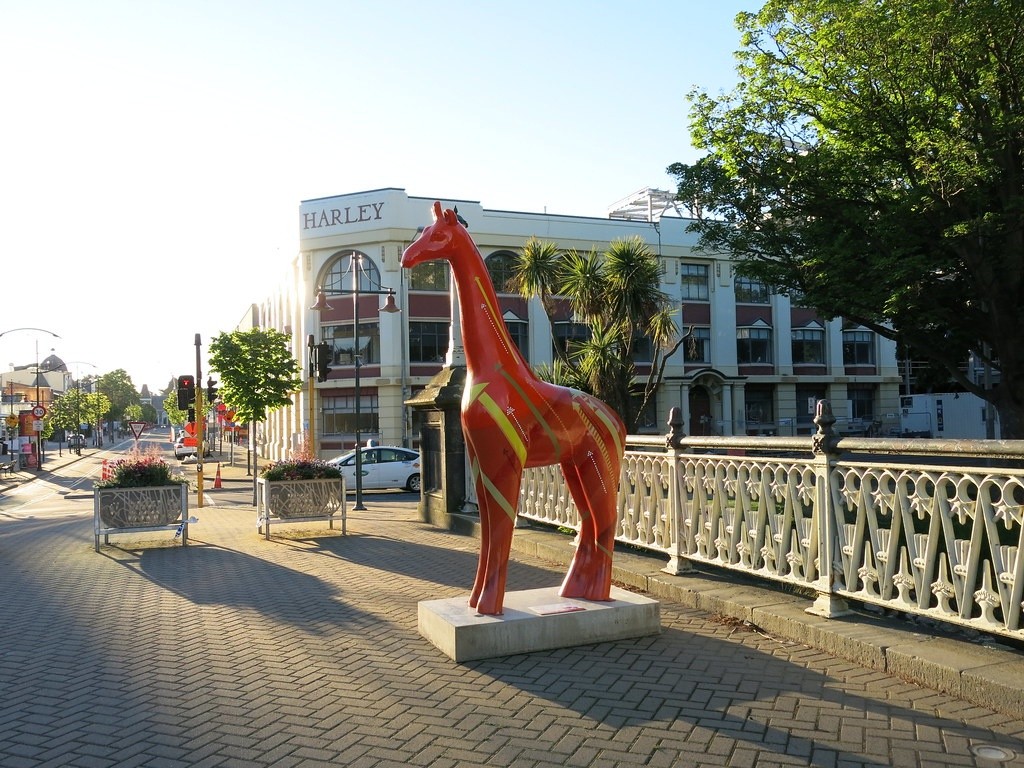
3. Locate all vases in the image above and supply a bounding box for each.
[100,486,182,529]
[261,481,342,520]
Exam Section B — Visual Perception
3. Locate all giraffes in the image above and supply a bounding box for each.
[399,199,626,617]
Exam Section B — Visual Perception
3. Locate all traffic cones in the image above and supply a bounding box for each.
[214,462,223,490]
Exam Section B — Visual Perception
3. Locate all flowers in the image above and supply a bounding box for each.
[259,456,342,481]
[94,445,199,493]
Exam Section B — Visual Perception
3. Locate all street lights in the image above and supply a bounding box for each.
[31,339,97,471]
[308,250,403,513]
[0,322,63,453]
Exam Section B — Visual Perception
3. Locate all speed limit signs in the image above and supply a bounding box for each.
[31,405,47,420]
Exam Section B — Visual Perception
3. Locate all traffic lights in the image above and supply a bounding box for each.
[207,376,219,404]
[187,408,195,423]
[178,376,196,403]
[315,344,334,383]
[178,388,187,410]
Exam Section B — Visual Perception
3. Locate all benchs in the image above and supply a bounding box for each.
[2,459,17,477]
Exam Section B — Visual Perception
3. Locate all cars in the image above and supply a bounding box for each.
[317,446,420,493]
[67,433,88,447]
[173,436,211,460]
